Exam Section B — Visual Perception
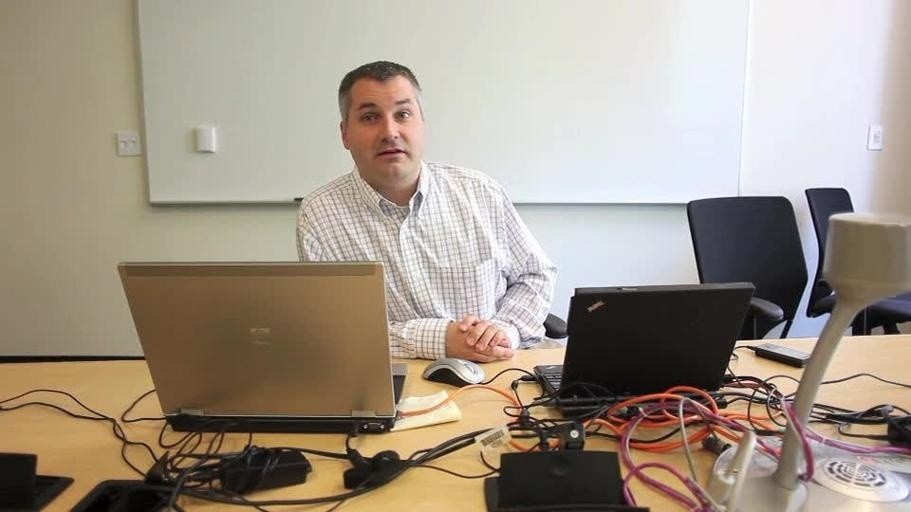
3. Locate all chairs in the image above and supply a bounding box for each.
[805,188,908,332]
[687,195,810,339]
[538,311,569,342]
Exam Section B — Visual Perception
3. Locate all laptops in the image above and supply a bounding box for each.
[118,260,409,435]
[534,282,756,417]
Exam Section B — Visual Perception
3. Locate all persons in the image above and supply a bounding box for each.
[297,60,557,365]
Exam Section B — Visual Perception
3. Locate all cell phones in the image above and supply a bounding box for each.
[757,343,812,368]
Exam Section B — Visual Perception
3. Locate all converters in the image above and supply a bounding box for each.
[220,446,309,494]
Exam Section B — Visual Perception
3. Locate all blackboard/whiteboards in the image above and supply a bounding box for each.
[131,0,750,207]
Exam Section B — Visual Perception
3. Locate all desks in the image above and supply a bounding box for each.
[1,332,911,510]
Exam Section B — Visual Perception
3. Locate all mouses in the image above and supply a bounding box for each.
[421,357,490,390]
